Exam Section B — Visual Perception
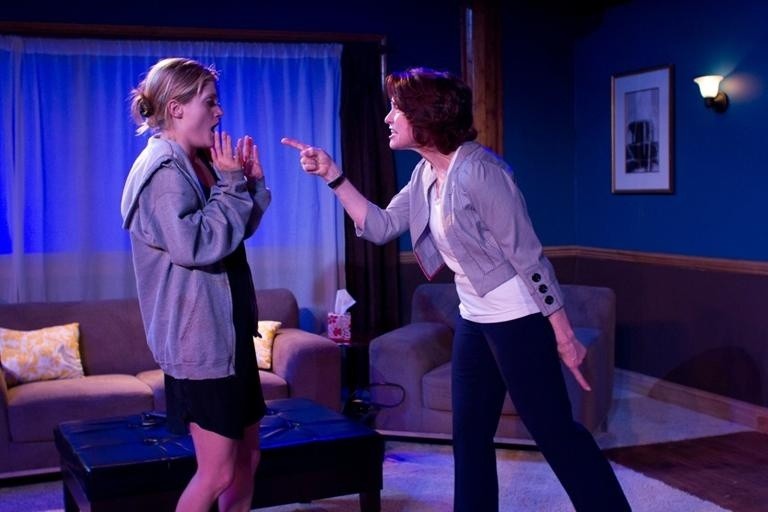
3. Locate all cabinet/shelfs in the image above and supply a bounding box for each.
[318,329,379,415]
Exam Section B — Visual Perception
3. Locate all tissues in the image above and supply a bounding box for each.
[327,288,357,343]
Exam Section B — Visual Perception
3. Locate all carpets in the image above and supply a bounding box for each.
[2,439,737,510]
[591,384,759,449]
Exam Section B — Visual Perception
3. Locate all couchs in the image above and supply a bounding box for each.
[0,287,344,483]
[365,280,617,452]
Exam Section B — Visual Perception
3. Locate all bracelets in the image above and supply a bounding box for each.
[327,170,347,190]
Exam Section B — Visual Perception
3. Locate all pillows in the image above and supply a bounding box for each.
[252,320,282,371]
[0,322,86,390]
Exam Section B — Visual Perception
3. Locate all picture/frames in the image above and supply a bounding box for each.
[607,61,678,197]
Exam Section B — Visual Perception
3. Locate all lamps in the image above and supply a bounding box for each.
[694,74,731,114]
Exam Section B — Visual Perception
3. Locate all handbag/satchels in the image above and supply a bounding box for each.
[342,383,406,429]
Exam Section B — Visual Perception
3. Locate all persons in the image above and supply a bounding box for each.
[120,57,271,512]
[280,67,632,511]
